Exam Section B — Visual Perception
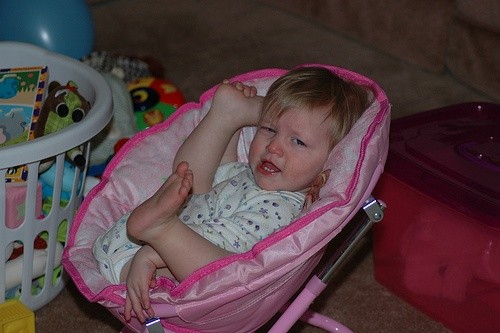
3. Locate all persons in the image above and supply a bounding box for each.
[93,66,371,324]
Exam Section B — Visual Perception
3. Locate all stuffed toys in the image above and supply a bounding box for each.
[32,80,86,174]
[80,49,150,82]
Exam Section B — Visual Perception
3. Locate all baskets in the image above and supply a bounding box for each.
[0,40,115,313]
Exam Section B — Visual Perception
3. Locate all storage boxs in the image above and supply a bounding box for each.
[0,301,35,333]
[373,101,500,333]
